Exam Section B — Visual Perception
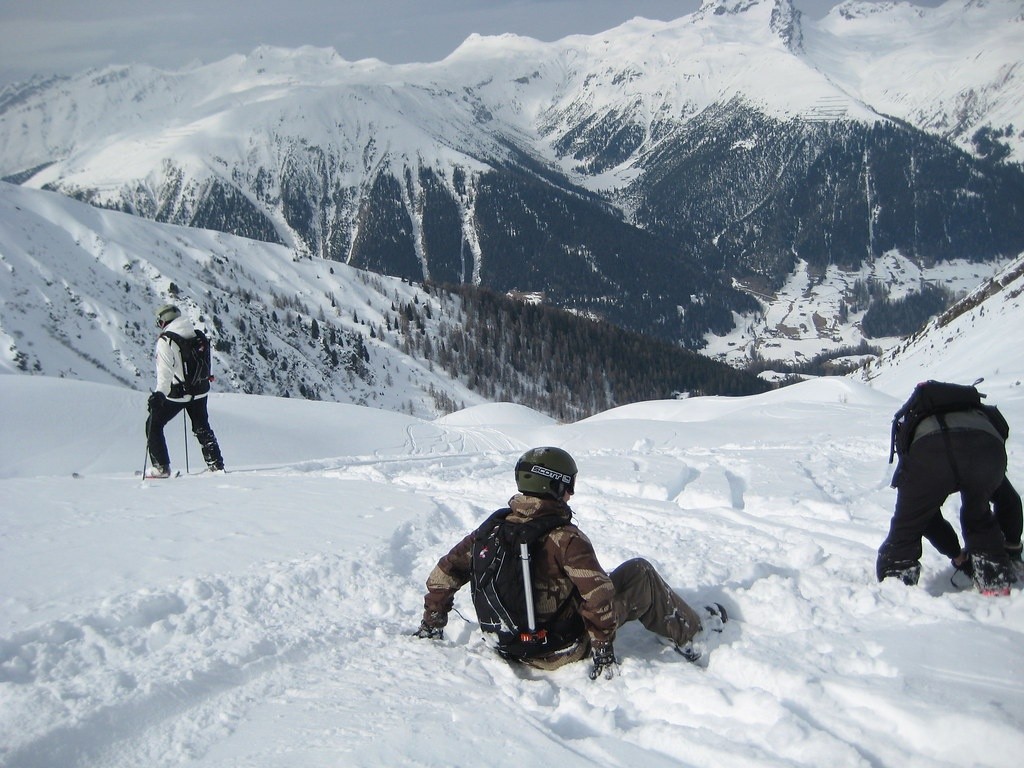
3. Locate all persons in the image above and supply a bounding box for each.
[876,380,1023,597]
[416,446,726,678]
[142,305,227,478]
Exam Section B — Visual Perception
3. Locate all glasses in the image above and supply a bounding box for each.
[157,319,164,327]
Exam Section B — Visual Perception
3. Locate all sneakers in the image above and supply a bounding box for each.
[145,466,170,478]
[973,552,1016,599]
[203,467,225,475]
[655,603,727,660]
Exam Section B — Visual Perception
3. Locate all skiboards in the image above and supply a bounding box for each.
[135,469,257,476]
[73,471,181,481]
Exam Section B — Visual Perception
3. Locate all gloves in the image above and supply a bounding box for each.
[586,645,624,681]
[1008,545,1023,567]
[412,621,443,639]
[147,392,167,408]
[950,552,973,575]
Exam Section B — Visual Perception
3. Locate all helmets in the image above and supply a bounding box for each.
[156,304,181,328]
[515,447,577,500]
